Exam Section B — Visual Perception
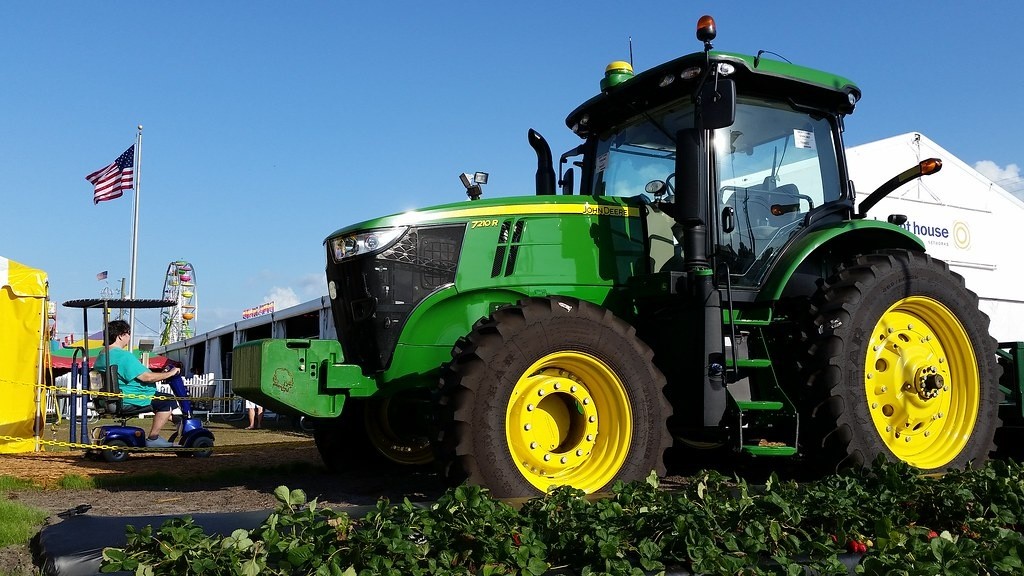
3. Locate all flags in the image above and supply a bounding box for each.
[96,271,108,281]
[84,143,135,205]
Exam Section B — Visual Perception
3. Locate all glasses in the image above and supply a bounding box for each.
[123,330,130,335]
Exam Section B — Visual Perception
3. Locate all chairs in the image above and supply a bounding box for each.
[725,181,799,259]
[96,364,137,414]
[189,385,216,421]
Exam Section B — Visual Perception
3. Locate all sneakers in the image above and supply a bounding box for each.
[145,436,173,448]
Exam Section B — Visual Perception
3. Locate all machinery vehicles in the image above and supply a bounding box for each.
[231,15,1024,505]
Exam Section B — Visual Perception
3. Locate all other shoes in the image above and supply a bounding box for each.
[245,426,255,430]
[257,425,262,429]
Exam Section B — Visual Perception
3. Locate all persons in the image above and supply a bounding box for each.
[92,319,180,447]
[244,399,264,430]
[185,367,204,398]
